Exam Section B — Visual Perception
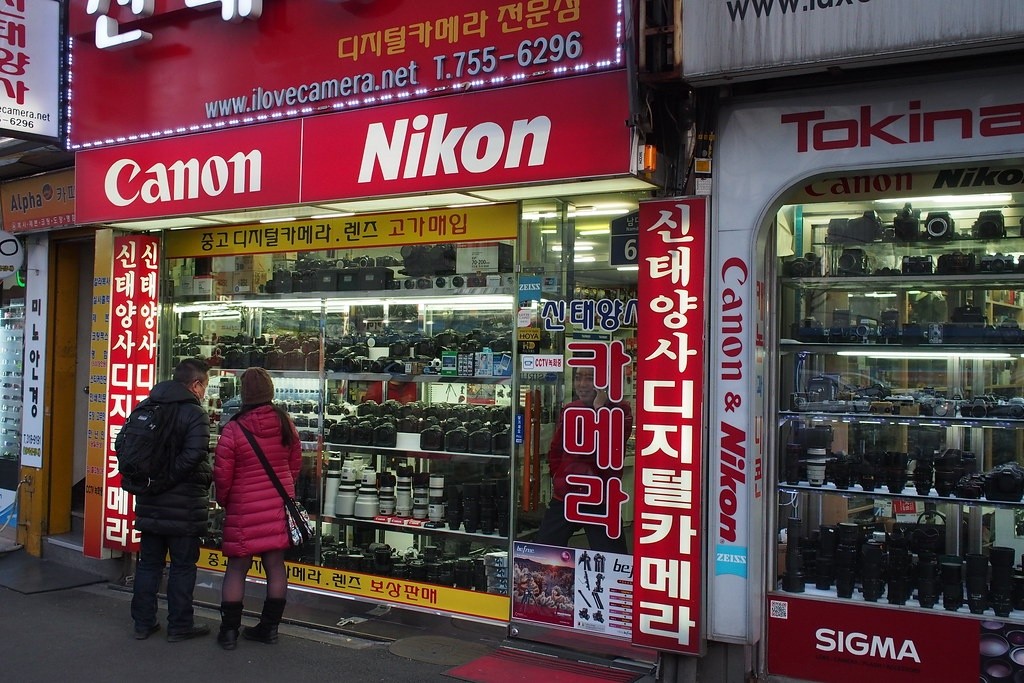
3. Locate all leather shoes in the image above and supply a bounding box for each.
[167,623,211,642]
[134,622,161,640]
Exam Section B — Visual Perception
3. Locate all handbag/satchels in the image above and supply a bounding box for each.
[284,499,316,547]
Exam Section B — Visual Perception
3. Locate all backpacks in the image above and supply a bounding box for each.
[115,401,203,497]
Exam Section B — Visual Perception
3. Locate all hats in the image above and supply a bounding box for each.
[241,367,274,405]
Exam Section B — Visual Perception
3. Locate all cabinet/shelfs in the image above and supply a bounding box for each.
[165,286,558,567]
[766,273,1024,625]
[822,291,1024,524]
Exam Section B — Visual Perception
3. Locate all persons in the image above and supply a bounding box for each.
[131,358,213,642]
[214,367,302,650]
[361,380,415,404]
[539,365,633,554]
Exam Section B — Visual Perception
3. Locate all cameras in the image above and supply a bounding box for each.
[780,203,1024,503]
[174,242,514,590]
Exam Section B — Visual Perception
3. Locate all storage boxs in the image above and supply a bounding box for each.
[316,269,337,291]
[487,587,507,594]
[337,268,360,291]
[483,552,509,567]
[455,241,514,275]
[179,276,193,295]
[232,271,272,294]
[235,254,273,272]
[359,267,395,290]
[215,271,235,294]
[485,566,508,578]
[486,576,508,588]
[193,275,215,295]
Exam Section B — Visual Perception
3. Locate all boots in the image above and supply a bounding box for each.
[217,599,244,650]
[242,597,287,644]
